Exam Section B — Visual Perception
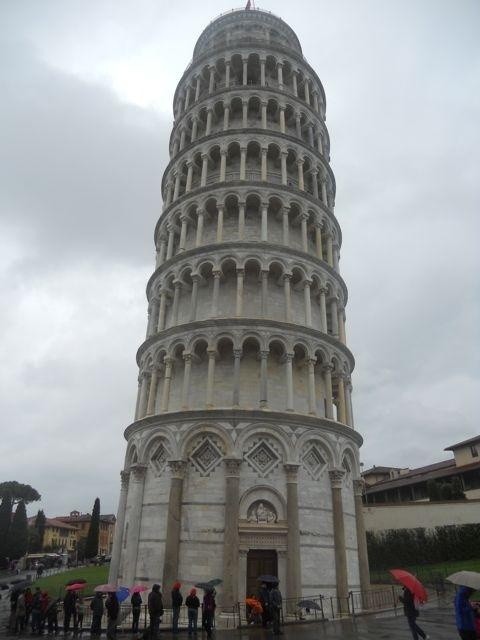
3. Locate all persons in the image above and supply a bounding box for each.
[395,583,431,640]
[471,599,479,636]
[453,586,479,640]
[5,571,285,637]
[306,608,310,615]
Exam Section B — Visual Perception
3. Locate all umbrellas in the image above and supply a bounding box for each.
[444,569,480,592]
[388,567,429,605]
[296,600,322,610]
